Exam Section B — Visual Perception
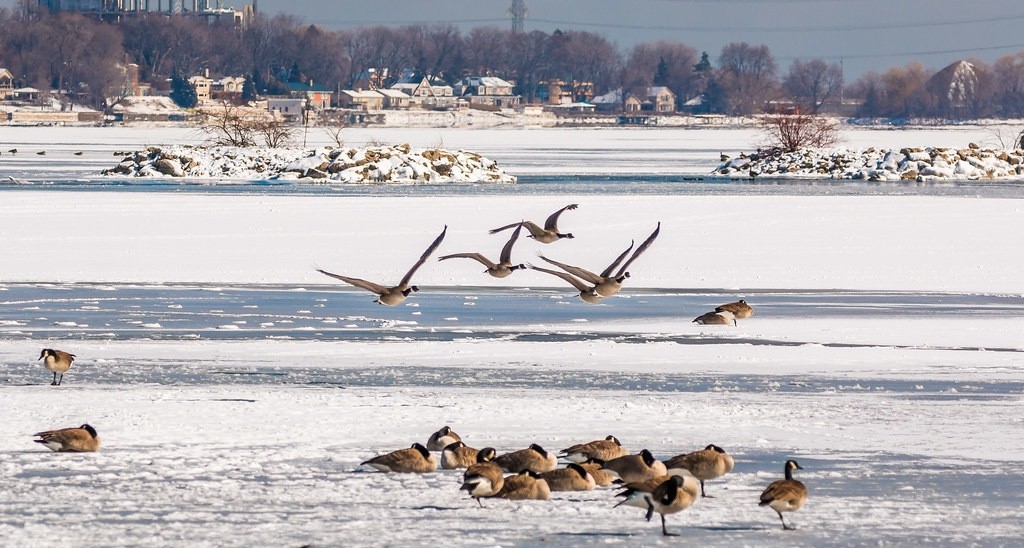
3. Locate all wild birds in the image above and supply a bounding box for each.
[38,348,77,387]
[719,151,746,160]
[427,426,735,535]
[438,220,527,279]
[488,204,579,244]
[758,460,807,531]
[9,149,17,156]
[316,224,448,307]
[691,299,755,325]
[359,442,439,472]
[32,424,100,453]
[526,221,660,304]
